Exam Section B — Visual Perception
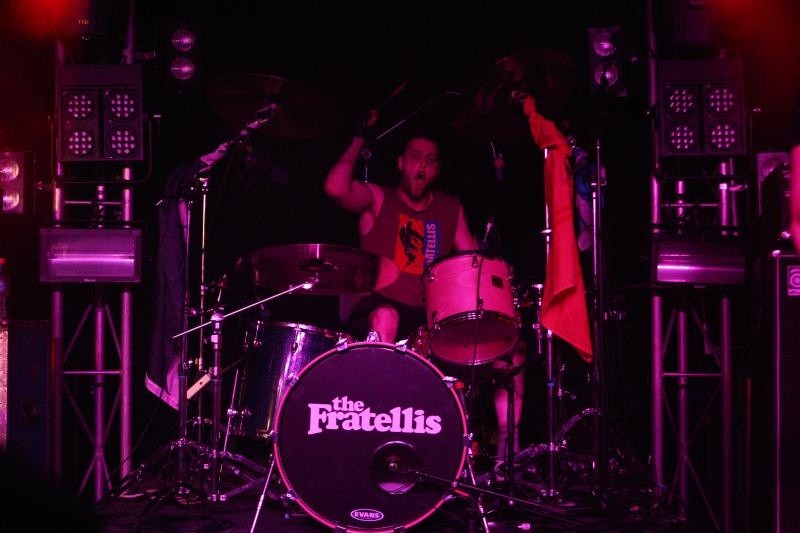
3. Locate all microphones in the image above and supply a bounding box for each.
[386,452,399,472]
[480,215,496,252]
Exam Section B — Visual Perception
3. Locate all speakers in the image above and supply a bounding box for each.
[762,251,800,533]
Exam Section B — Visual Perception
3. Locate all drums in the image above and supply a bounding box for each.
[215,320,355,441]
[527,281,627,368]
[272,340,469,533]
[419,250,524,366]
[407,321,528,388]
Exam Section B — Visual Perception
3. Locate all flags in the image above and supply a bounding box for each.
[522,96,593,364]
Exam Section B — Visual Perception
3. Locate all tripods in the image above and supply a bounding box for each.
[460,138,675,520]
[84,111,300,533]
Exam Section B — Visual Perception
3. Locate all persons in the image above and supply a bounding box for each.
[325,54,606,485]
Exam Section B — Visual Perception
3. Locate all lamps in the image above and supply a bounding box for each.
[594,62,617,87]
[173,26,196,52]
[593,28,614,57]
[170,56,195,80]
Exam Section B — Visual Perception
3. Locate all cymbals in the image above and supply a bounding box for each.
[235,244,399,296]
[536,97,656,133]
[206,73,350,139]
